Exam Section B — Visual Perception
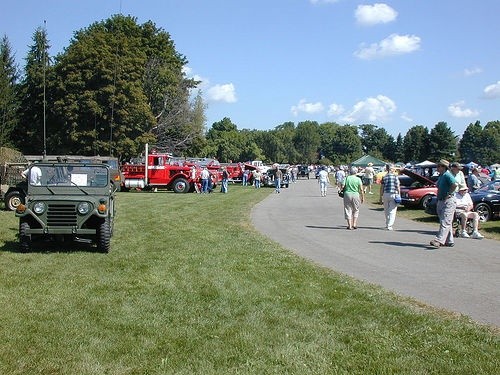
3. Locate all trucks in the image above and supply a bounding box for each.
[119,140,253,197]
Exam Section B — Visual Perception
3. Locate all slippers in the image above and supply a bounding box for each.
[347,226,353,230]
[353,226,357,229]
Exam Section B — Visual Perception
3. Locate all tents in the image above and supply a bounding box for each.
[413,160,438,177]
[351,154,385,168]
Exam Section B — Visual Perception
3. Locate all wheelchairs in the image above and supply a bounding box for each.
[453,213,485,240]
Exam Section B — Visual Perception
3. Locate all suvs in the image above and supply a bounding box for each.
[13,155,119,254]
[296,165,310,180]
[0,154,74,212]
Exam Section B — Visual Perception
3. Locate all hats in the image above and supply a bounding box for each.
[451,162,464,170]
[436,159,450,169]
[367,162,374,167]
[458,184,469,191]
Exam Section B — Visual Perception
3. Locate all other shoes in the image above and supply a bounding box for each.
[472,232,485,239]
[369,192,374,195]
[364,189,366,194]
[448,242,455,247]
[430,241,440,248]
[461,230,470,238]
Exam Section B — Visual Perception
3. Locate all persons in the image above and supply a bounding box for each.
[200,167,209,193]
[21,160,42,186]
[273,166,283,194]
[253,170,261,188]
[429,159,484,247]
[468,167,481,191]
[243,169,249,186]
[317,165,348,197]
[363,163,374,195]
[342,165,364,230]
[291,168,297,184]
[380,163,401,231]
[221,167,229,193]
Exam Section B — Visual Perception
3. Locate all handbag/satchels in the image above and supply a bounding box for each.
[394,196,402,204]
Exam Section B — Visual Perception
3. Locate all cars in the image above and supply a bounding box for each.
[262,166,290,190]
[392,160,500,223]
[315,167,330,179]
